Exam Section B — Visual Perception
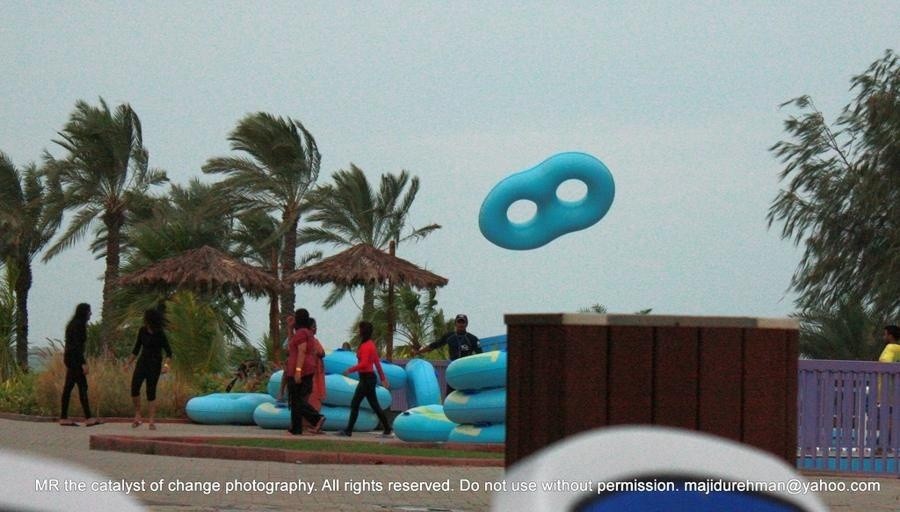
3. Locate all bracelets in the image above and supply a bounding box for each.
[164,364,169,368]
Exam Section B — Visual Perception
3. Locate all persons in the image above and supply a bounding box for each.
[410,315,483,398]
[123,310,172,430]
[877,326,900,407]
[343,343,351,349]
[334,320,393,437]
[278,309,326,435]
[59,303,99,426]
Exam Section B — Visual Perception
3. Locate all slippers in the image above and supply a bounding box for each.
[61,421,80,427]
[87,420,104,426]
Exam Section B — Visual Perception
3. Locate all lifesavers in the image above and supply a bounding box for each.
[478,151,615,251]
[253,347,508,443]
[186,392,276,425]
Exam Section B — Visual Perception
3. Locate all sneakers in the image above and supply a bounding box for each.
[132,421,156,431]
[336,431,352,436]
[381,428,393,435]
[287,416,326,435]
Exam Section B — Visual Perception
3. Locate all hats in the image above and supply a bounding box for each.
[455,314,467,321]
